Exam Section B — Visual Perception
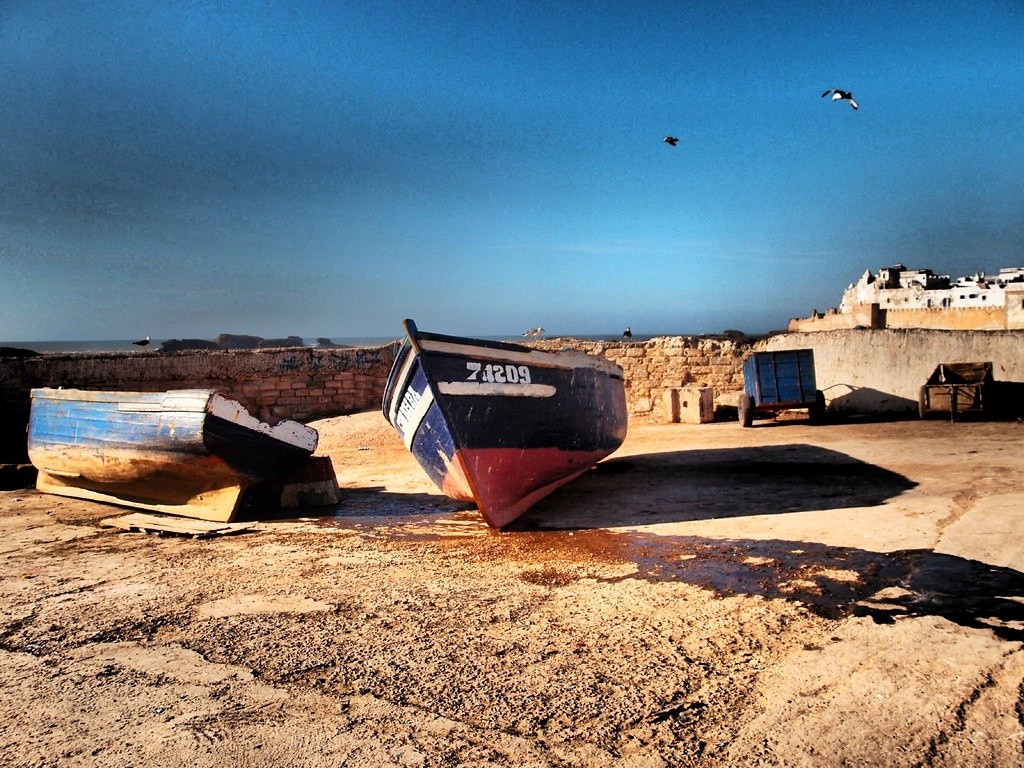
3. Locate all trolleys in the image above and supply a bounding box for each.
[737,348,828,427]
[917,362,999,423]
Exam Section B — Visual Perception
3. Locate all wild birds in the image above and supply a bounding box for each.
[822,89,859,110]
[523,327,545,341]
[663,136,678,147]
[623,327,632,342]
[132,337,152,352]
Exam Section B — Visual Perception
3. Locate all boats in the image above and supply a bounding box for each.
[379,317,631,530]
[25,387,320,527]
